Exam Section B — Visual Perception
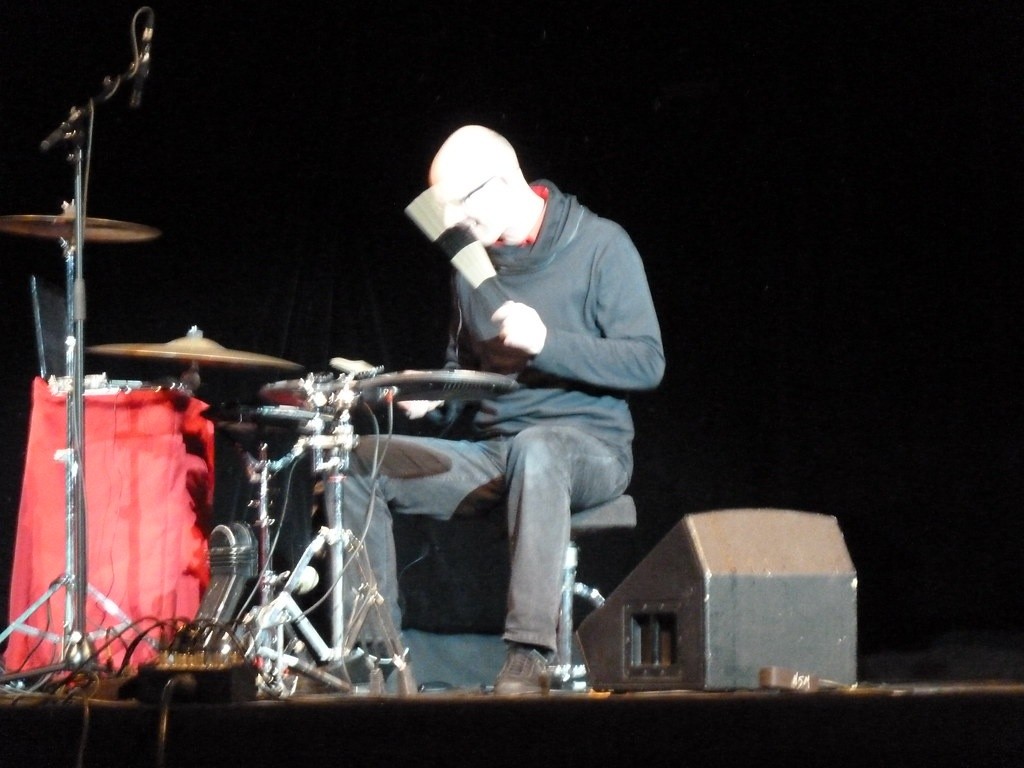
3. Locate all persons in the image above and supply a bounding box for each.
[323,124,665,695]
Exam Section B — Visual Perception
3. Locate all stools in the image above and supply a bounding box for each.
[478,495,636,691]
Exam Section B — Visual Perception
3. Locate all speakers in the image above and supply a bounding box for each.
[577,507,858,691]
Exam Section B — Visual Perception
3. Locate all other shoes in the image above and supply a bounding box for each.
[494,646,550,694]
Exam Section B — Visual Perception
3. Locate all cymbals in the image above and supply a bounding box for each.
[83,338,306,372]
[0,213,161,245]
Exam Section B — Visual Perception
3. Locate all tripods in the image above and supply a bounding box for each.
[238,409,418,704]
[0,57,169,693]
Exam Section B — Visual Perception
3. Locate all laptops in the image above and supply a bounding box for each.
[29,272,68,380]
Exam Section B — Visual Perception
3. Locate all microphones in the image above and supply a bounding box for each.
[127,13,154,107]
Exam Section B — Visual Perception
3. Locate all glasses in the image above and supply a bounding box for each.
[454,177,493,208]
